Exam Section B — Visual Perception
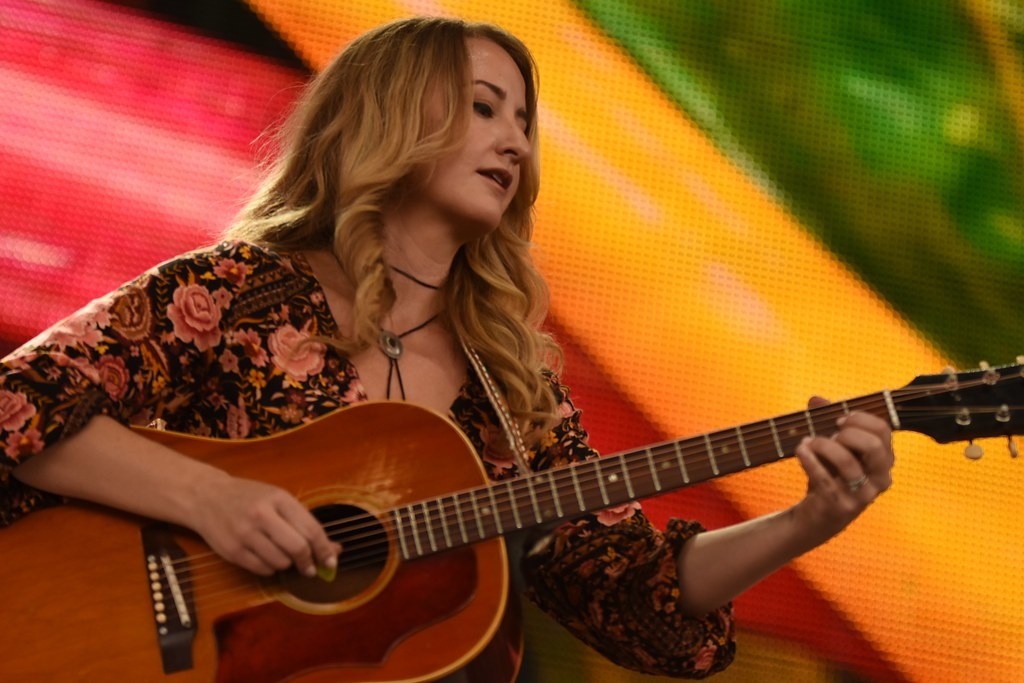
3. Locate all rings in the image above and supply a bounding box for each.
[852,474,869,490]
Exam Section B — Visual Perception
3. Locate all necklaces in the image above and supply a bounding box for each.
[332,245,445,403]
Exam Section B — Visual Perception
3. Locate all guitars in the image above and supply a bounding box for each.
[0,336,1024,683]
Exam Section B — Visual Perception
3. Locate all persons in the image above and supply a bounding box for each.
[0,16,893,683]
